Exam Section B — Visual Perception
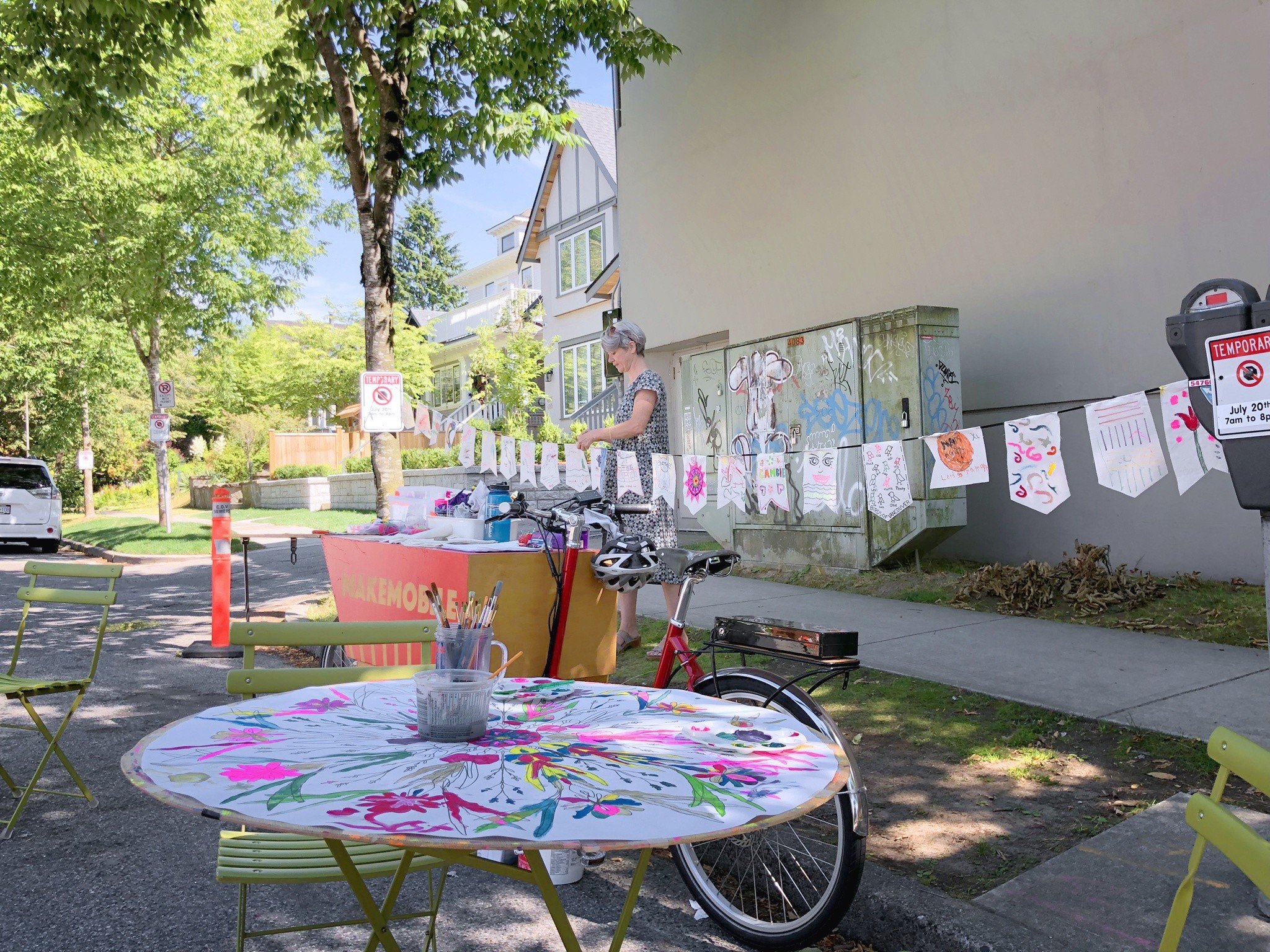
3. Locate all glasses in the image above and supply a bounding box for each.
[607,325,637,344]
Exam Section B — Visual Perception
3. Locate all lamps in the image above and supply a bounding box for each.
[544,368,553,382]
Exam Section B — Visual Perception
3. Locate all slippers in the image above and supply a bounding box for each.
[646,636,692,662]
[617,629,641,655]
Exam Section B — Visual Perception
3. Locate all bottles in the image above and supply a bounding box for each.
[483,481,512,543]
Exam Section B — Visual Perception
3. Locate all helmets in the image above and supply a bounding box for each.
[590,533,657,593]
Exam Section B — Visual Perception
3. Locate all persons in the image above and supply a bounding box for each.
[576,320,689,660]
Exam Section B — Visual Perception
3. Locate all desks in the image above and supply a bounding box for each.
[120,681,849,952]
[223,530,617,682]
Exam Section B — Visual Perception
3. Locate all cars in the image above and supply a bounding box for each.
[0,454,62,554]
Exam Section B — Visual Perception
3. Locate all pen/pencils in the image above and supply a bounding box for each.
[518,679,575,692]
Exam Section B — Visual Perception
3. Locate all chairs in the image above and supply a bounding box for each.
[215,621,450,952]
[0,560,123,840]
[1159,726,1270,952]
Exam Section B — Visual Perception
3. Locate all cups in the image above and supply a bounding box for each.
[435,623,508,693]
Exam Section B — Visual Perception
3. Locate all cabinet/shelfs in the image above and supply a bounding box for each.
[680,305,968,570]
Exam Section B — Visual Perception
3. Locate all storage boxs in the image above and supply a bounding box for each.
[387,485,522,541]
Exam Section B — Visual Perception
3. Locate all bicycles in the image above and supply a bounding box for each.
[485,492,870,952]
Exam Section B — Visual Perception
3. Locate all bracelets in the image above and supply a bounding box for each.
[609,426,612,441]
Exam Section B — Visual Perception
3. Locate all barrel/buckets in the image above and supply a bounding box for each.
[412,669,498,743]
[567,526,589,549]
[513,849,584,885]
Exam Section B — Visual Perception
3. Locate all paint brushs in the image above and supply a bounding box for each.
[456,580,504,670]
[486,651,523,680]
[425,582,454,669]
